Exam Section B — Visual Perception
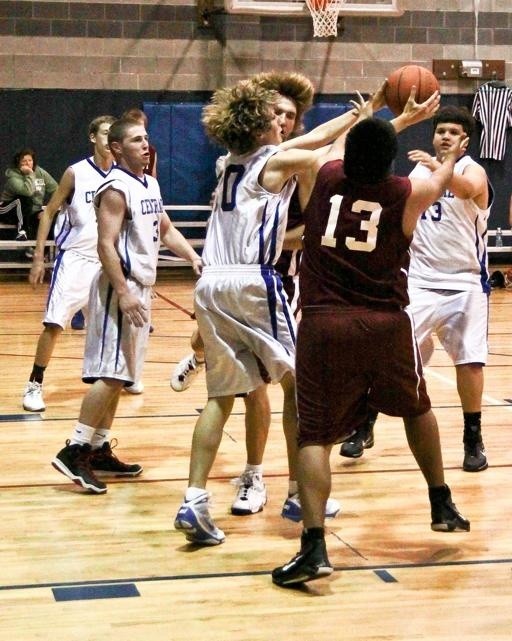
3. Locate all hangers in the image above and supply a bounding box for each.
[472,67,512,95]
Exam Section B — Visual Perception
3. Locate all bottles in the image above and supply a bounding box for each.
[495,228,503,247]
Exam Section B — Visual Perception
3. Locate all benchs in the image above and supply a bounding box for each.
[487,230,512,252]
[0,205,213,276]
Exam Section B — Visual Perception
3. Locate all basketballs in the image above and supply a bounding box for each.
[387,65,439,117]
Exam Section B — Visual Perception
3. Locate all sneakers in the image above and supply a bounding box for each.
[16,230,27,241]
[340,431,374,457]
[126,381,144,393]
[52,439,142,494]
[272,491,340,585]
[431,504,470,532]
[231,471,267,514]
[463,441,488,471]
[171,354,206,391]
[23,381,45,411]
[174,493,225,545]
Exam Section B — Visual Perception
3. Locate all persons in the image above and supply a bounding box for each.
[1,148,60,260]
[174,71,441,548]
[338,105,489,474]
[23,107,157,410]
[51,118,203,494]
[272,90,471,586]
